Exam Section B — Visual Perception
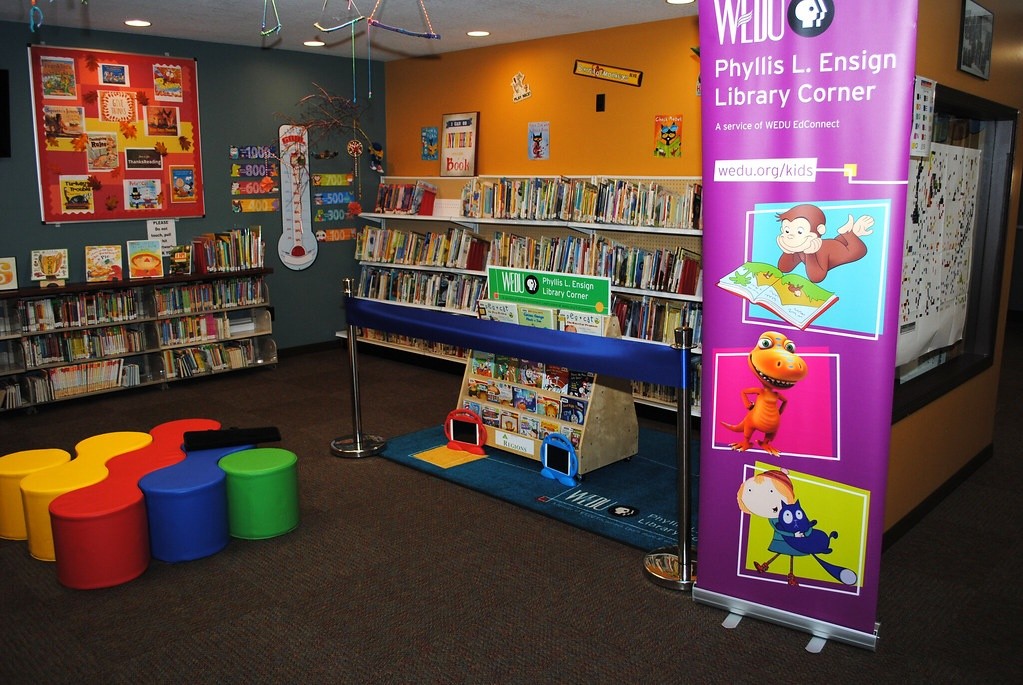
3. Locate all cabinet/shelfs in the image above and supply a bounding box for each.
[440,265,639,482]
[333,173,703,426]
[0,266,275,423]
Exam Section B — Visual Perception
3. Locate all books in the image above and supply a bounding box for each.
[348,173,702,453]
[0,225,277,409]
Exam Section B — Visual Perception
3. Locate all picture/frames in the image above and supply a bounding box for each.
[650,112,683,159]
[438,109,480,179]
[525,119,551,160]
[420,125,440,161]
[956,0,995,80]
[26,41,207,224]
